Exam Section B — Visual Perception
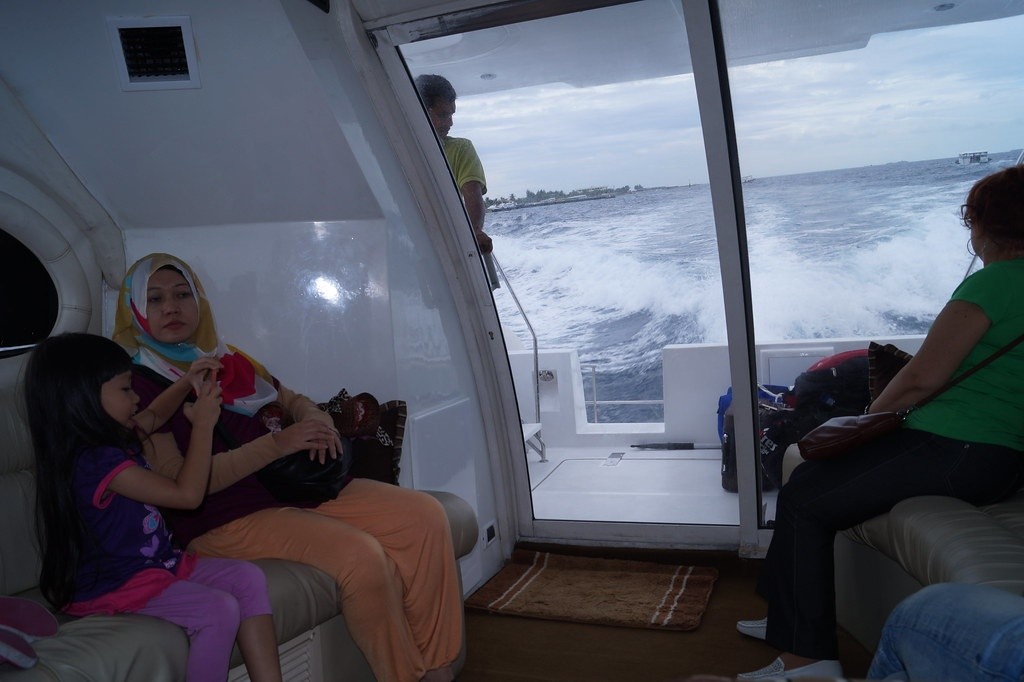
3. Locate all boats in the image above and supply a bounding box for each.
[954,151,992,164]
[741,175,756,183]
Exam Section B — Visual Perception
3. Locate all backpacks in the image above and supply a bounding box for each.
[723,400,793,493]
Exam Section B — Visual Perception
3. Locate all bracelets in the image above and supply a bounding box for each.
[864,403,869,415]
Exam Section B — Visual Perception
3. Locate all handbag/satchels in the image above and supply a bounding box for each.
[247,402,354,508]
[796,409,907,459]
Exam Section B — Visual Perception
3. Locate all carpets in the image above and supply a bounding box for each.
[466,545,718,632]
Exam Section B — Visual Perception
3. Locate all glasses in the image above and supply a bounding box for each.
[962,217,976,229]
[426,104,456,120]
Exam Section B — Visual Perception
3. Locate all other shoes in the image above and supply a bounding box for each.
[0,628,39,669]
[736,617,767,640]
[0,594,59,638]
[737,656,844,682]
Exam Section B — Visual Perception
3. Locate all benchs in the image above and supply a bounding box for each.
[783,445,1024,656]
[1,379,480,681]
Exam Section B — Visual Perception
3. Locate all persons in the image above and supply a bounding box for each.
[112,253,470,682]
[22,332,283,681]
[865,581,1024,682]
[412,74,494,256]
[738,165,1024,682]
